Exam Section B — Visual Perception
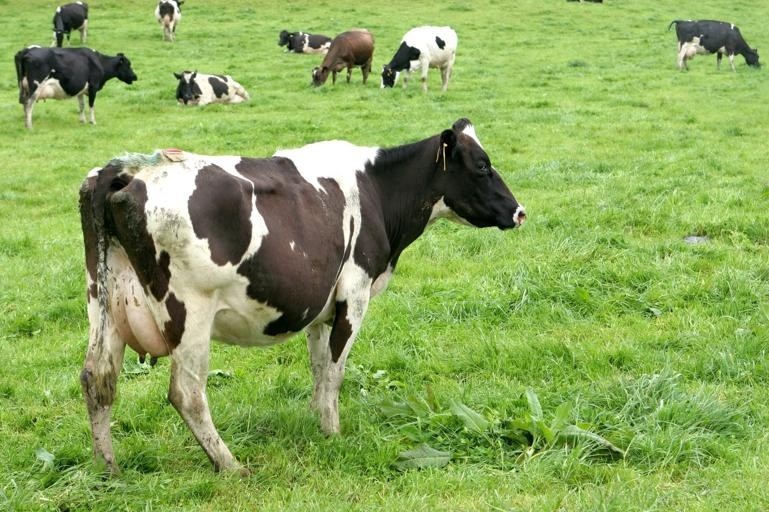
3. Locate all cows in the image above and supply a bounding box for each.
[78,117,526,484]
[668,18,760,74]
[16,44,137,131]
[154,0,185,41]
[278,30,333,55]
[51,1,89,48]
[173,69,250,109]
[312,28,375,89]
[379,26,459,97]
